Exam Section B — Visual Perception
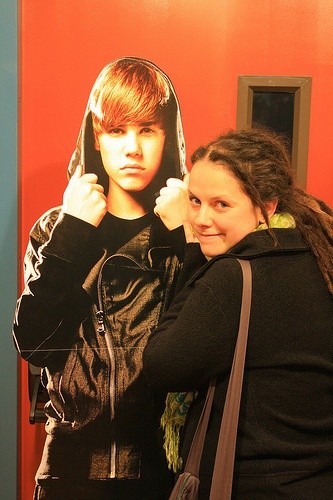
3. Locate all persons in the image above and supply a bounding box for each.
[12,57,212,500]
[143,130,333,500]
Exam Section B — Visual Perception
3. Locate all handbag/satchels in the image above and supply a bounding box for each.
[169,472,199,500]
